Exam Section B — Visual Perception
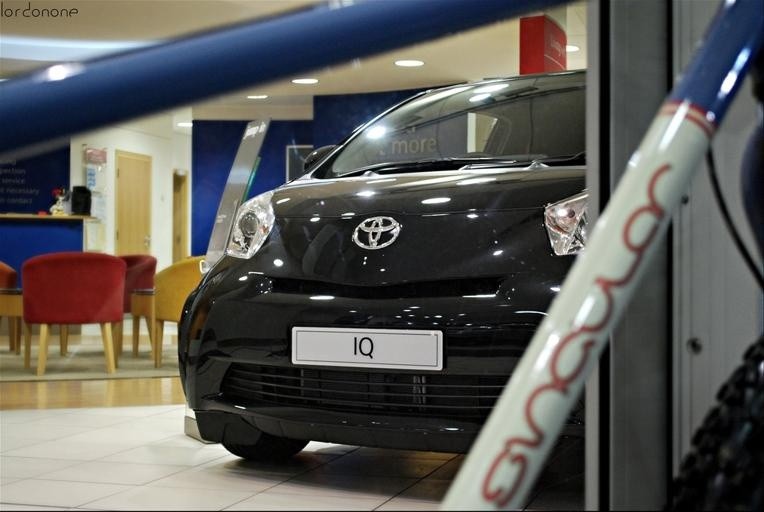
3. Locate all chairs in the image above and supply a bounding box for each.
[1,254,204,375]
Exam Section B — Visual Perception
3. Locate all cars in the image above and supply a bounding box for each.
[176,67,588,466]
